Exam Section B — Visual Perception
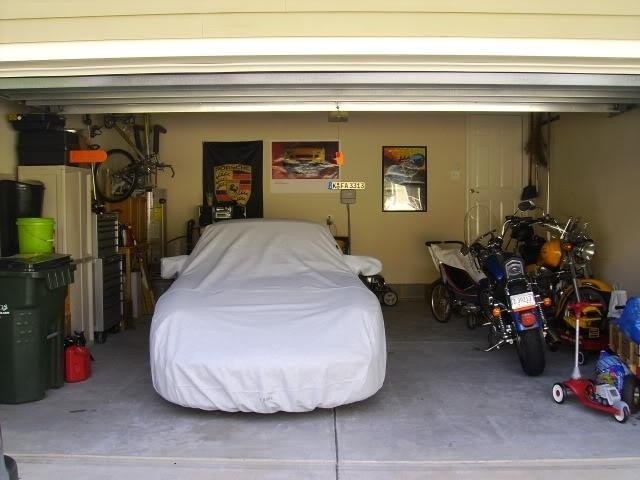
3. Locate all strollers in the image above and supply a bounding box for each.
[358,274,398,307]
[425,240,487,329]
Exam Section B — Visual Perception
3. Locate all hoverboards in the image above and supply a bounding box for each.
[553,301,630,424]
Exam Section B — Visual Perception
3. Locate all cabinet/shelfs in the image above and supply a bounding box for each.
[106,189,166,278]
[92,212,124,333]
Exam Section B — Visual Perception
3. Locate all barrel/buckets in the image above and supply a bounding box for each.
[15,217,56,254]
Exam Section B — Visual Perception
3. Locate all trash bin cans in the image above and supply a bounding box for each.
[151,271,174,301]
[0,253,76,404]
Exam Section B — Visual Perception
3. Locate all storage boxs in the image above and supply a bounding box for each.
[608,324,640,374]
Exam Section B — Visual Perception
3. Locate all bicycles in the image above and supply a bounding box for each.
[89,112,176,203]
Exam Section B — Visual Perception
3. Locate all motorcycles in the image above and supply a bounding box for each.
[460,200,562,377]
[503,214,617,358]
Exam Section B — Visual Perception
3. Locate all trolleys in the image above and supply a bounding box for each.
[545,265,616,367]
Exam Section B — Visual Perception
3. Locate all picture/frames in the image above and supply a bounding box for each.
[382,145,427,213]
